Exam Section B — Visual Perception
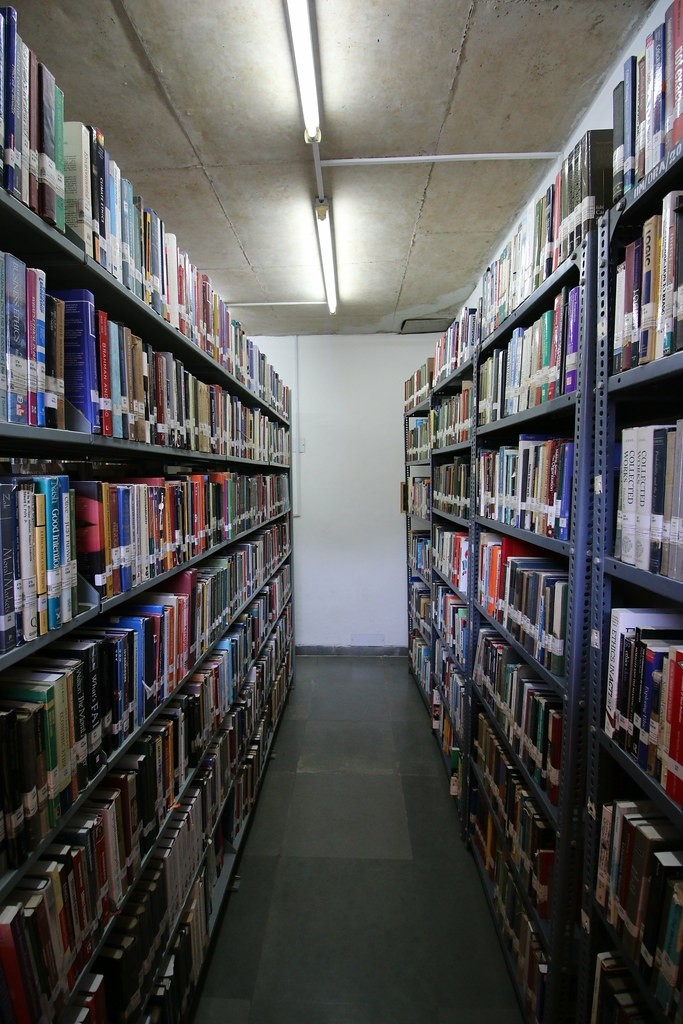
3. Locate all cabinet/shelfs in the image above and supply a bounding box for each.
[407,139,683,1024]
[1,186,296,1024]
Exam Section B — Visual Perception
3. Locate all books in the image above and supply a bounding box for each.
[406,4,683,1024]
[0,4,296,1024]
